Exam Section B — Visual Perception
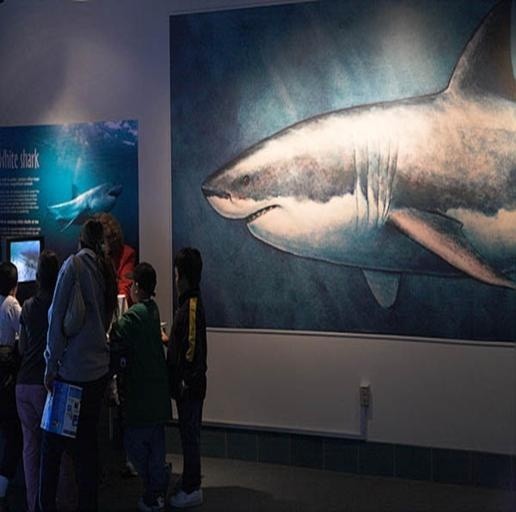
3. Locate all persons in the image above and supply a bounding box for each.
[1,208,208,511]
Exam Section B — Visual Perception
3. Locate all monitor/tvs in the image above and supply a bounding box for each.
[6,236,45,285]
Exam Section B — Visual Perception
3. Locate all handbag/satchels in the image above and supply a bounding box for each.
[63,282,86,336]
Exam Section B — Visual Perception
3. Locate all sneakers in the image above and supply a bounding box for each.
[168,490,203,508]
[138,494,165,510]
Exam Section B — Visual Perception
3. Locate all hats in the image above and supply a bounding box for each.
[124,262,157,294]
